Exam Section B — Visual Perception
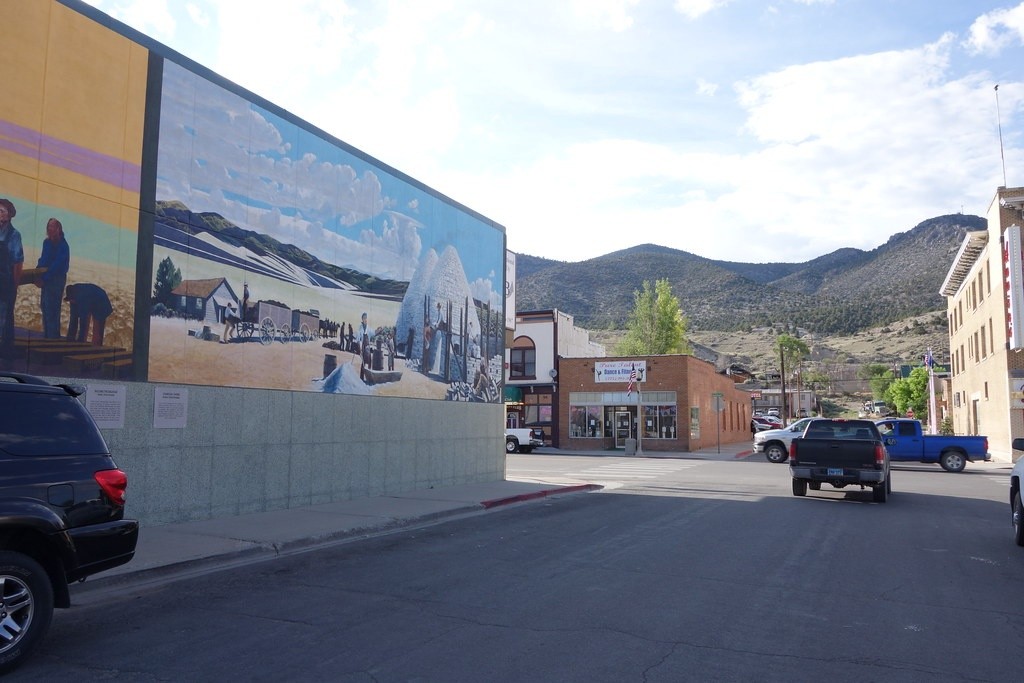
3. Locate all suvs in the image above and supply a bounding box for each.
[750,417,816,463]
[796,409,808,418]
[768,407,780,416]
[0,374,140,668]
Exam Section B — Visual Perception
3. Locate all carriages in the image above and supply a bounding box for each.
[236,298,340,345]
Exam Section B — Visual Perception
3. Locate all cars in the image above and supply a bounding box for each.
[1009,437,1024,545]
[755,410,764,415]
[752,415,782,432]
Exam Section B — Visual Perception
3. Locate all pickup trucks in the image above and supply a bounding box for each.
[506,428,544,453]
[789,418,890,502]
[871,420,992,472]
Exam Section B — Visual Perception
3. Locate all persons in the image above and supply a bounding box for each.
[884,423,893,435]
[907,407,914,417]
[751,420,756,441]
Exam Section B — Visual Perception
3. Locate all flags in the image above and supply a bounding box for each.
[925,352,933,369]
[627,369,636,397]
